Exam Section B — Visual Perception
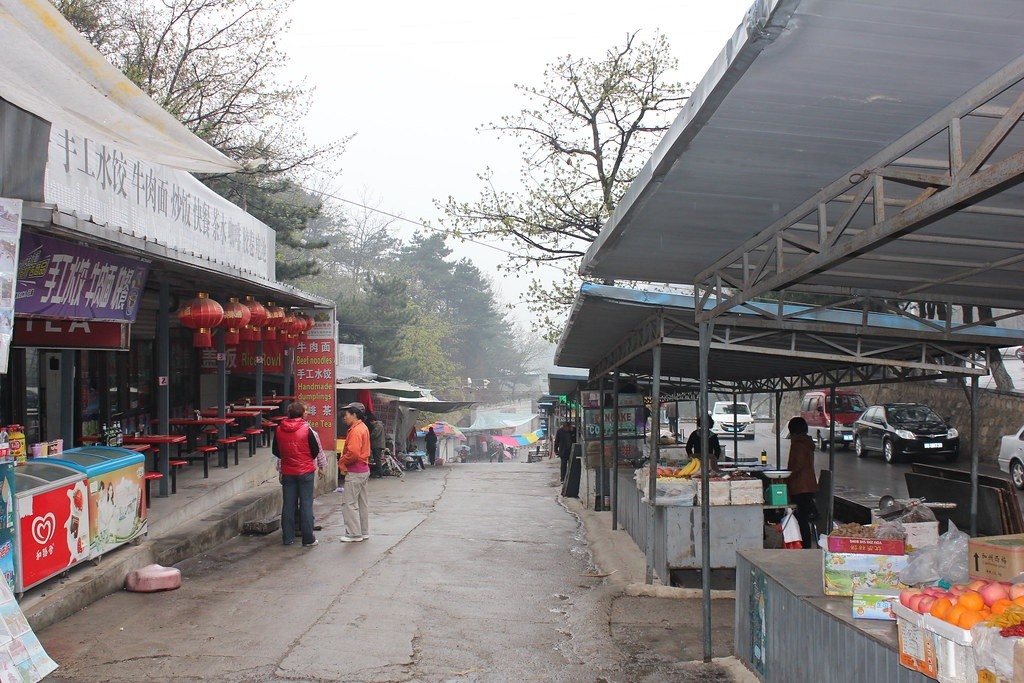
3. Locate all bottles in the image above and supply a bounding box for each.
[194,410,200,420]
[0,428,10,462]
[9,432,26,465]
[246,399,251,403]
[100,421,123,447]
[230,403,234,413]
[138,424,144,436]
[761,451,766,463]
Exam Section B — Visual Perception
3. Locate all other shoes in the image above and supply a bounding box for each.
[303,540,318,546]
[294,528,302,536]
[313,525,321,530]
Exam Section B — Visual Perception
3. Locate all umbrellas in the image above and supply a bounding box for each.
[454,445,471,452]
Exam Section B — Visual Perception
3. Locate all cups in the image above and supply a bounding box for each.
[31,439,63,460]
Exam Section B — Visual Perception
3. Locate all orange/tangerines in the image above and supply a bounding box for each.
[930,590,1024,630]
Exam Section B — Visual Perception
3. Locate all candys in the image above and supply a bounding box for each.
[6,424,24,432]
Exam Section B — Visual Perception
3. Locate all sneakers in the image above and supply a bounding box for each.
[362,534,369,539]
[340,536,363,542]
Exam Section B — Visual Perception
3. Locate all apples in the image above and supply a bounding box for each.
[657,468,682,476]
[900,580,1024,613]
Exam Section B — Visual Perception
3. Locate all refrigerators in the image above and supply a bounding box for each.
[27,446,148,566]
[0,462,90,600]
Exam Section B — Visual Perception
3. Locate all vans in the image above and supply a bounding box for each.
[800,390,870,452]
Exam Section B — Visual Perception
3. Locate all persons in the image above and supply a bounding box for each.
[458,447,467,463]
[424,427,437,465]
[685,414,721,462]
[497,442,504,462]
[95,481,120,532]
[363,409,385,479]
[337,402,371,541]
[414,445,419,451]
[783,416,817,549]
[553,421,581,484]
[271,399,327,547]
[665,401,680,432]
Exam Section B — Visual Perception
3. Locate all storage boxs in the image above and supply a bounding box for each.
[637,469,764,505]
[820,509,1024,682]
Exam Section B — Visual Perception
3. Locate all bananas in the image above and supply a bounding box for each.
[678,457,701,475]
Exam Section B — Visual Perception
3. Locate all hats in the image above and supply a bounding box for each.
[340,402,365,414]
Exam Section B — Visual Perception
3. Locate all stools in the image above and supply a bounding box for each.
[145,416,287,508]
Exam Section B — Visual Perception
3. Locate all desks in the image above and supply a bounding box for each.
[79,395,295,474]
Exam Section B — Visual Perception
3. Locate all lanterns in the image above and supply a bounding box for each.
[219,297,251,345]
[240,295,316,346]
[178,292,224,348]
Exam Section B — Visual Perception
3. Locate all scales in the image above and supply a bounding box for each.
[762,470,792,506]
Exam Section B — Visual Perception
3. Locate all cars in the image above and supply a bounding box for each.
[707,401,756,440]
[998,425,1024,491]
[852,402,960,465]
